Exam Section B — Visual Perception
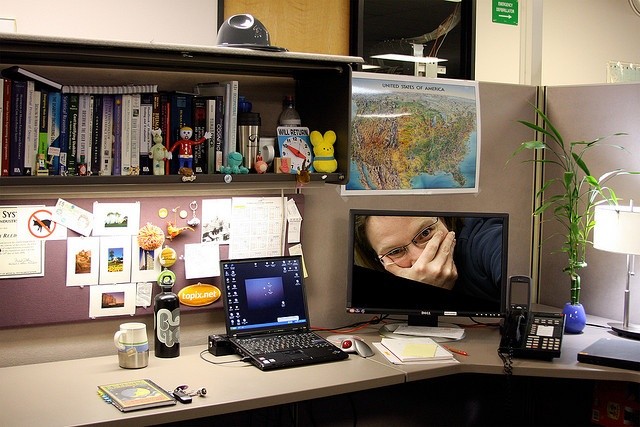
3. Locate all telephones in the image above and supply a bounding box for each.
[497,306,566,377]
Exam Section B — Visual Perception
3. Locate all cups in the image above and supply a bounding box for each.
[259,136,275,163]
[113,323,150,353]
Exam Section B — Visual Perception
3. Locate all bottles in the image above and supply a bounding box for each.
[279,94,301,126]
[153,275,180,357]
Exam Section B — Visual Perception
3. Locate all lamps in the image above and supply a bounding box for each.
[367,39,450,65]
[592,197,640,337]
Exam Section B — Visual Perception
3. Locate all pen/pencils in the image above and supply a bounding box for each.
[441,345,467,356]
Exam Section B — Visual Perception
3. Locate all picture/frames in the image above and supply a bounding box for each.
[273,125,317,175]
[67,201,164,319]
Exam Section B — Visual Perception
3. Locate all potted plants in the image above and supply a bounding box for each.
[506,98,640,336]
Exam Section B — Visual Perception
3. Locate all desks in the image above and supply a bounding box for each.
[1,303,640,427]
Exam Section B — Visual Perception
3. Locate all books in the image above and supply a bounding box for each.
[0,67,239,176]
[371,338,460,364]
[590,382,639,426]
[97,379,177,413]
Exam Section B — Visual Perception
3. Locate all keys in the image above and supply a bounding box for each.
[168,384,207,404]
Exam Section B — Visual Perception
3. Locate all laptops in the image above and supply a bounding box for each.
[219,256,349,370]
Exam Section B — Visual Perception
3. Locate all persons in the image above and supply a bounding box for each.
[354,215,499,302]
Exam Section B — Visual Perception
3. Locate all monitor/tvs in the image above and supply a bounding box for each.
[348,207,510,336]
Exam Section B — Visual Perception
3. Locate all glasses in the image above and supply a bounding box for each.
[375,216,438,264]
